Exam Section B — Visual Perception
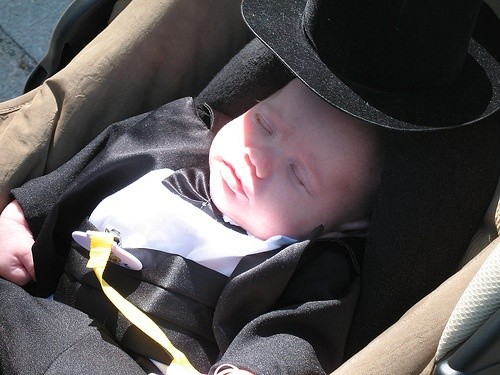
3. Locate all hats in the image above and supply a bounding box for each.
[241,0,499,132]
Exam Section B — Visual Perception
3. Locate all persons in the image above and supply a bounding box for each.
[2,77,395,375]
